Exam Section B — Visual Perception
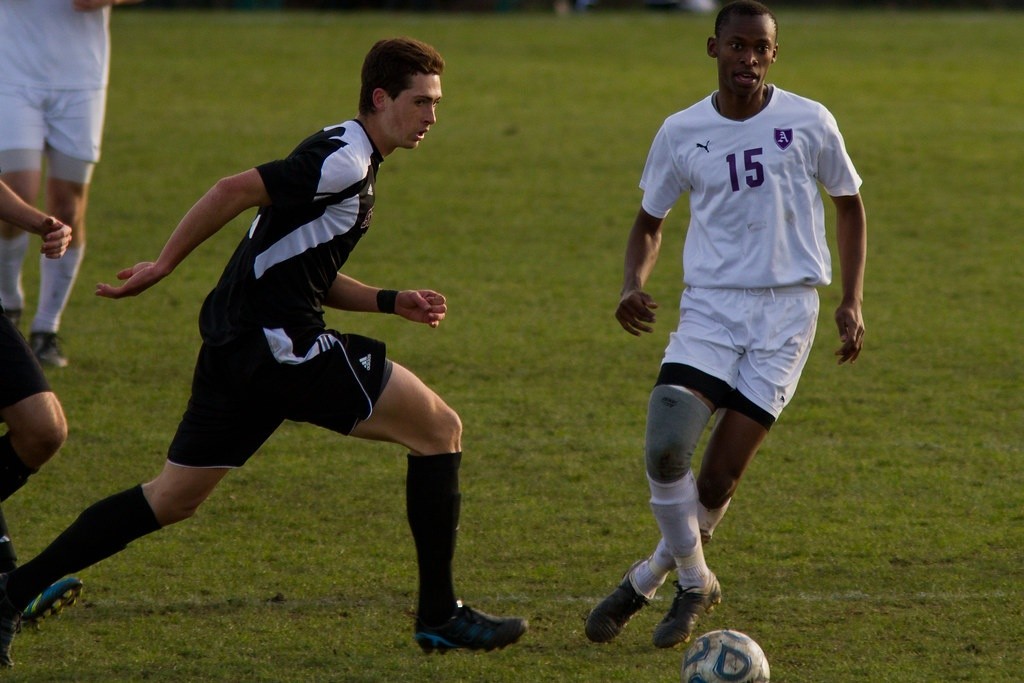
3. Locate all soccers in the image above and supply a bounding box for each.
[681,628,770,683]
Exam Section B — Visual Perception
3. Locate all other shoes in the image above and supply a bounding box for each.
[1,309,21,327]
[29,332,68,368]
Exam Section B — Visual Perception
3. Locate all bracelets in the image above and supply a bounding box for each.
[376,289,397,314]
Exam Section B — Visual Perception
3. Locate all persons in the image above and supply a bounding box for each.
[0,37,527,668]
[583,0,867,646]
[0,182,84,633]
[0,0,137,369]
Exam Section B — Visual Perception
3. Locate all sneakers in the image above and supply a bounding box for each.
[585,560,657,644]
[415,599,528,655]
[20,577,83,624]
[0,573,23,666]
[653,573,722,650]
